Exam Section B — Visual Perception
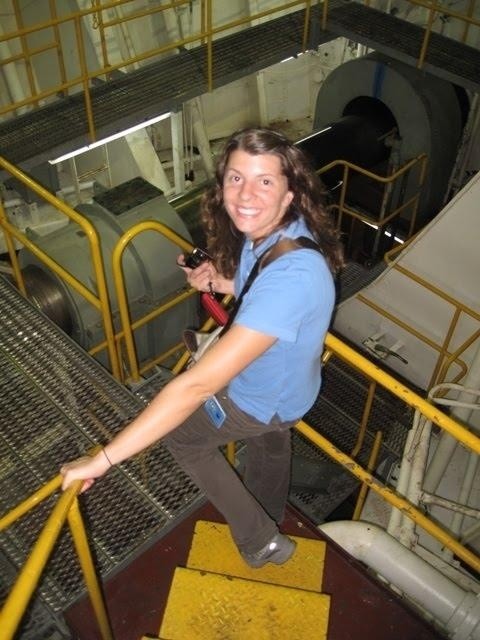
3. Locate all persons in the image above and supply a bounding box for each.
[56,126,350,572]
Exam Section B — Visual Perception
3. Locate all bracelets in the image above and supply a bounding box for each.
[101,446,115,468]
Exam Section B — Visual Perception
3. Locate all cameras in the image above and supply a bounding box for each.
[185,244,217,275]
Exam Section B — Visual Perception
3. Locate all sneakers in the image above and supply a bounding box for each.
[237,528,297,570]
